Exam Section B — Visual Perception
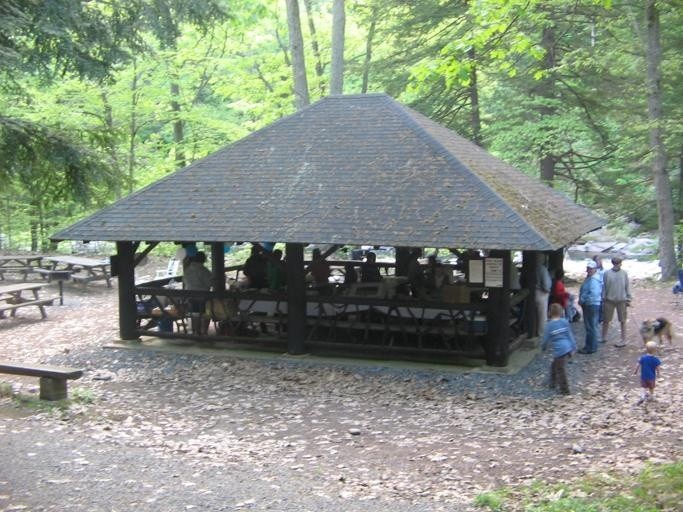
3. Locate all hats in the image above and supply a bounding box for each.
[587,261,597,268]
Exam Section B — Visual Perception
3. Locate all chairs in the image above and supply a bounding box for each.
[125,255,522,371]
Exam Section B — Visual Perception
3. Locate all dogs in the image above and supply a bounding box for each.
[638,316,673,352]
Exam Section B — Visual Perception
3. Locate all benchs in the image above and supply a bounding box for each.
[1,358,86,400]
[1,254,117,324]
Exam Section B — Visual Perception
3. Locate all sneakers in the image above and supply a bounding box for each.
[614,342,624,347]
[597,337,608,343]
[578,348,589,354]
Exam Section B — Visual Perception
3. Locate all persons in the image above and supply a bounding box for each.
[456,248,480,275]
[535,253,632,395]
[180,243,449,341]
[635,340,662,406]
[510,257,522,294]
